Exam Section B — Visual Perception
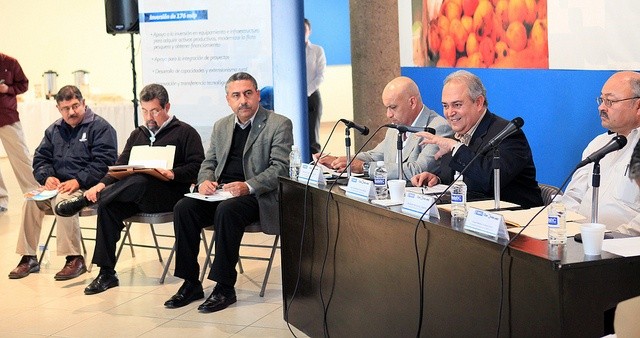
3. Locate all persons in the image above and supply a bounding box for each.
[163,72,294,312]
[562,70,640,239]
[304,19,327,162]
[409,69,543,211]
[316,76,454,179]
[54,83,205,298]
[9,85,118,279]
[0,52,39,210]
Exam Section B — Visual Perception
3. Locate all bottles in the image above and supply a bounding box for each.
[547,195,568,245]
[374,160,388,201]
[289,146,301,180]
[449,173,467,217]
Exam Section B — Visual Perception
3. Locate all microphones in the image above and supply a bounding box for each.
[341,119,369,135]
[385,124,435,135]
[480,117,524,153]
[575,135,627,169]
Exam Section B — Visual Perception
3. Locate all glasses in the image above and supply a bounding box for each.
[596,95,640,108]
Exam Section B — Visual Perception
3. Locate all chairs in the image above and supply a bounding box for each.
[38,206,98,272]
[111,210,212,284]
[200,222,280,297]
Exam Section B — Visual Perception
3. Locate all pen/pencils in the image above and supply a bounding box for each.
[421,189,424,193]
[215,186,223,189]
[423,184,428,190]
[309,151,332,164]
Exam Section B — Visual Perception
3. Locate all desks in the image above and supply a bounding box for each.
[277,169,640,338]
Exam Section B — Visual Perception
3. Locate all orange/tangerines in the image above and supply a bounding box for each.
[426,0,547,67]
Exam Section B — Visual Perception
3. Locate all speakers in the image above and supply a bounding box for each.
[105,0,139,34]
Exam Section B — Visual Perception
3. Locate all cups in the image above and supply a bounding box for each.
[579,222,606,255]
[388,179,406,204]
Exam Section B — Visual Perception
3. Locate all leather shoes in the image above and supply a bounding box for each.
[164,281,205,308]
[54,257,87,280]
[198,286,237,312]
[9,254,40,278]
[84,271,119,294]
[55,195,87,217]
